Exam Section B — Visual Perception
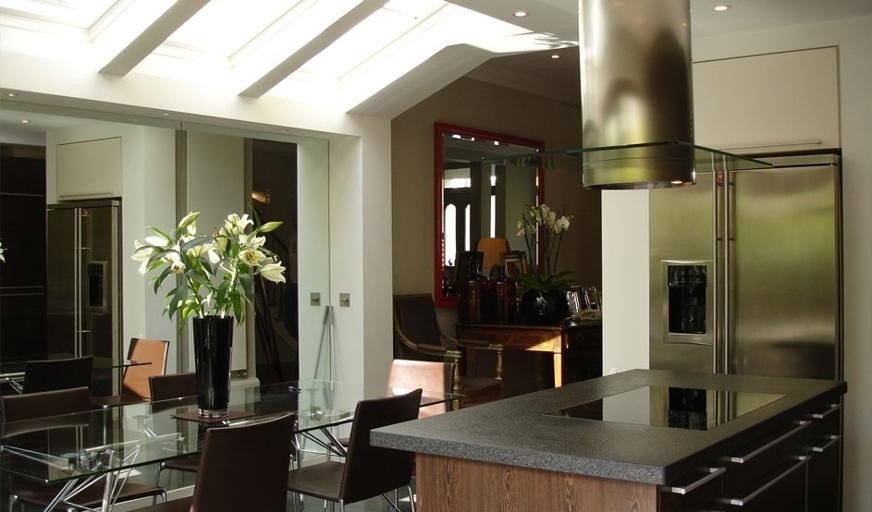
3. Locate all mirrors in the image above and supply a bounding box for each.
[1,100,330,512]
[434,121,548,306]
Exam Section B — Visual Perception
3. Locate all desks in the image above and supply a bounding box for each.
[450,316,601,391]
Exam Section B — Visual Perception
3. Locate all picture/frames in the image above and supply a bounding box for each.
[454,251,485,283]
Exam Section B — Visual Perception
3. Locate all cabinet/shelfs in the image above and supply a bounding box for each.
[658,398,843,512]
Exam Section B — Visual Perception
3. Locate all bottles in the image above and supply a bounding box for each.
[493,276,518,326]
[458,275,489,324]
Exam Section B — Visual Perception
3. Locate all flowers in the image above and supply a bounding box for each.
[130,203,290,319]
[519,199,578,294]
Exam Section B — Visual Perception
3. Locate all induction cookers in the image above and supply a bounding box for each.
[548,384,783,432]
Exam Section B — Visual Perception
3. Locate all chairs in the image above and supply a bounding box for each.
[289,387,423,511]
[0,337,294,512]
[391,289,505,414]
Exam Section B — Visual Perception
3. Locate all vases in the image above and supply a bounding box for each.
[191,314,234,414]
[517,288,567,324]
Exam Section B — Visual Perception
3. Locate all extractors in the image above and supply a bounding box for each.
[468,0,775,194]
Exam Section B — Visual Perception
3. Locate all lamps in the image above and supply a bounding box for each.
[577,0,699,190]
[477,236,512,281]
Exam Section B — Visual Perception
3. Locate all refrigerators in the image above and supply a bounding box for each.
[649,147,842,379]
